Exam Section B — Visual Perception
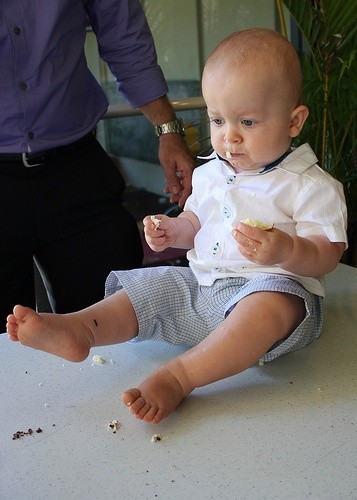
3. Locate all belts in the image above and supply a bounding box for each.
[0,129,96,168]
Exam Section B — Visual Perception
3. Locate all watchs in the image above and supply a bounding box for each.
[154,118,185,138]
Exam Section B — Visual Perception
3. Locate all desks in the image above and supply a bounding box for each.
[0,262,357,500]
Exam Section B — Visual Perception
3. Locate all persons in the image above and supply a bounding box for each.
[6,28,349,424]
[0,0,197,333]
